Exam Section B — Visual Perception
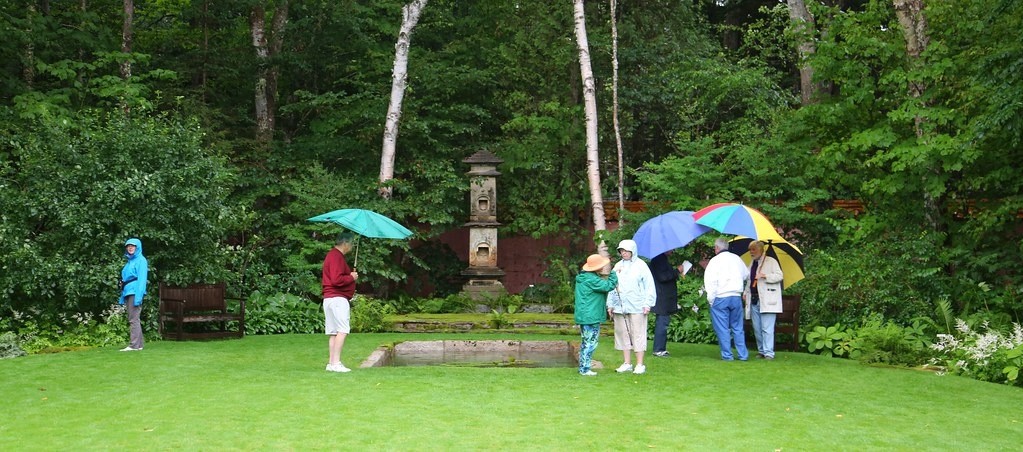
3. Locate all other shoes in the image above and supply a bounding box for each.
[579,368,597,375]
[653,351,670,358]
[755,353,773,360]
[325,362,351,373]
[119,344,142,352]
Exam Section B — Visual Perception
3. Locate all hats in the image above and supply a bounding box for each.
[582,253,611,271]
[337,232,356,243]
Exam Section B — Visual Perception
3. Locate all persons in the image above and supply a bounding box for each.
[117,238,148,350]
[606,240,656,374]
[743,240,783,358]
[703,238,749,361]
[650,249,685,357]
[319,232,360,369]
[572,254,618,375]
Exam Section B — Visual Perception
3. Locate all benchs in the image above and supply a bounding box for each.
[744,294,800,351]
[159,281,245,341]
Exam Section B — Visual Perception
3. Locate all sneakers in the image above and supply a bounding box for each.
[615,362,632,373]
[633,365,645,374]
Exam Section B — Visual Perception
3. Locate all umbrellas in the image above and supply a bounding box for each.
[691,201,778,252]
[306,207,414,272]
[726,233,805,290]
[630,210,714,262]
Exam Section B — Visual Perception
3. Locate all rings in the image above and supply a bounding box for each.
[357,275,358,276]
[356,272,357,275]
